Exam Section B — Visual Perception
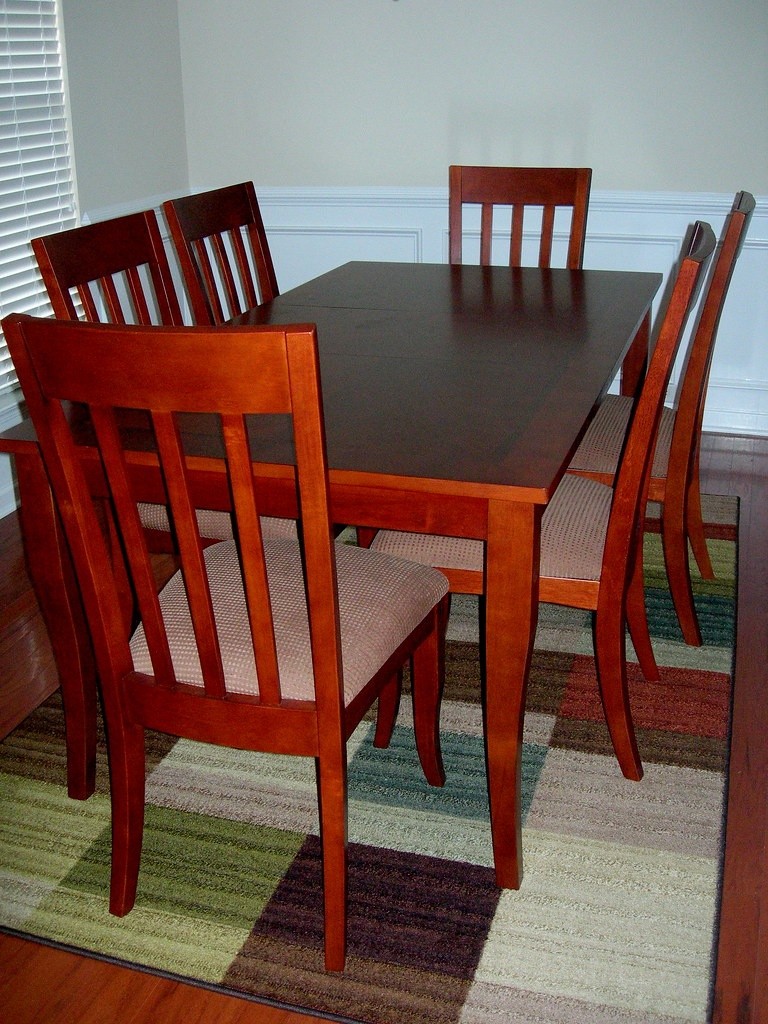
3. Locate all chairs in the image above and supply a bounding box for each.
[562,190,755,647]
[158,181,279,326]
[28,210,358,638]
[447,164,592,270]
[357,220,715,789]
[0,312,454,971]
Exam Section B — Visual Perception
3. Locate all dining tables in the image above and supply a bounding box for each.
[0,262,662,891]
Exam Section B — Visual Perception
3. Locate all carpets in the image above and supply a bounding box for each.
[1,491,740,1024]
